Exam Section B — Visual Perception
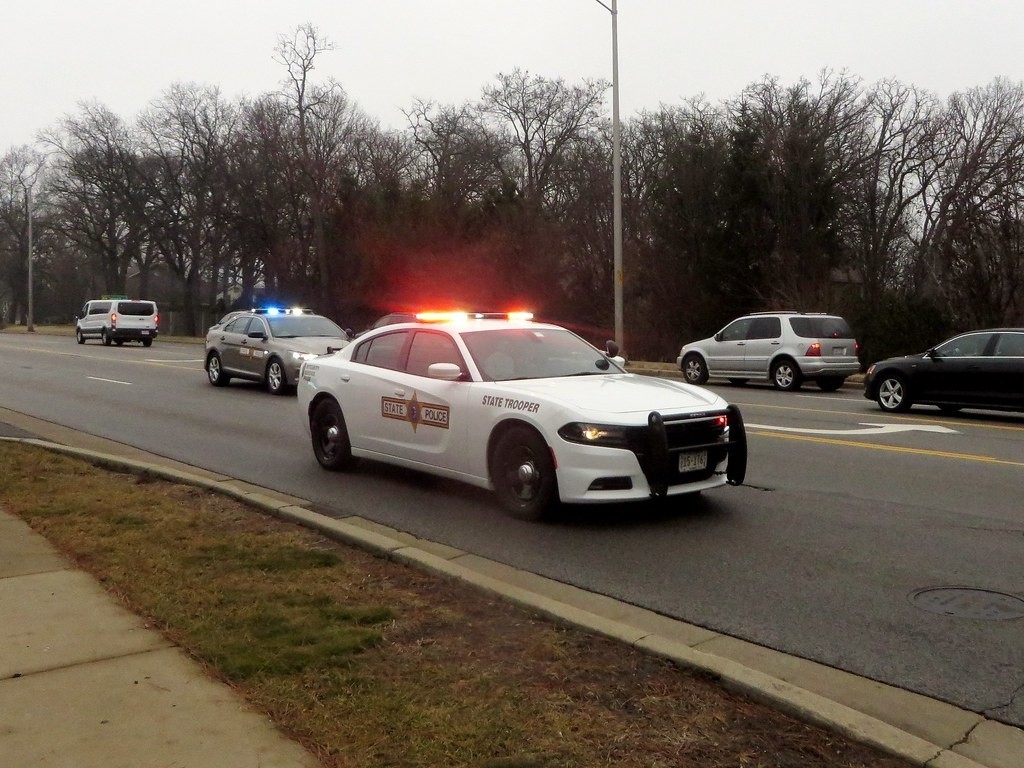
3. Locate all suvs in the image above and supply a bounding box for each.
[676,309,860,389]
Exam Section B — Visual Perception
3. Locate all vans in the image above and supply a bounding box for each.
[75,300,160,345]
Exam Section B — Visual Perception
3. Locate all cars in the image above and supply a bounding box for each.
[355,313,509,338]
[209,311,247,331]
[204,309,356,395]
[863,328,1024,411]
[297,312,748,518]
